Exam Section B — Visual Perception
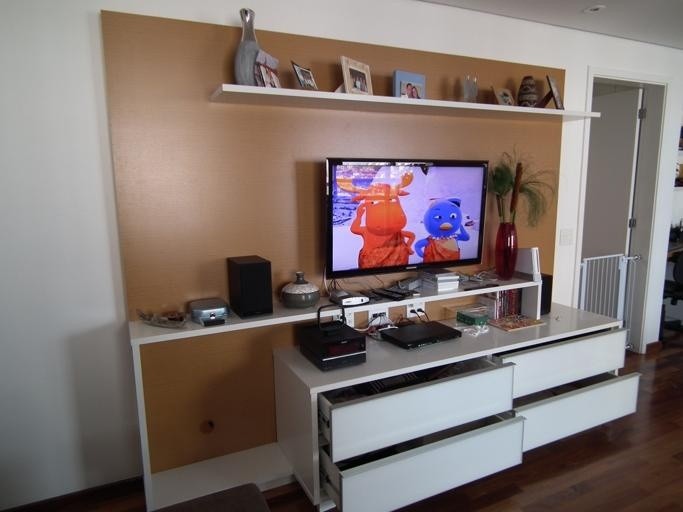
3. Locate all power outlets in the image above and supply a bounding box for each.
[405,302,424,318]
[368,308,389,325]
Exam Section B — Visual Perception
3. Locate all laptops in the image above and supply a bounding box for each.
[381,320,462,349]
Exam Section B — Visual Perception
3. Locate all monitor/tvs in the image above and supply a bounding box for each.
[325,157,489,280]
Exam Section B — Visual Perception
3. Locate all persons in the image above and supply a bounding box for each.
[413,87,420,98]
[351,72,367,91]
[406,83,413,98]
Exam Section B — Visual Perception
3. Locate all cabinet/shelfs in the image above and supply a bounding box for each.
[125,275,641,511]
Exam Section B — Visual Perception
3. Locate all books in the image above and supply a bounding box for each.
[479,289,545,332]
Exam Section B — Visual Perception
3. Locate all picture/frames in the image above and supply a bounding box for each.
[339,55,372,94]
[535,74,564,110]
[489,85,517,107]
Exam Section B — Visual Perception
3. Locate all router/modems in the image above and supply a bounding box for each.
[329,289,369,307]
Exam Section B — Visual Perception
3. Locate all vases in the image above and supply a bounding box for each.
[279,270,322,310]
[234,7,266,87]
[492,221,517,279]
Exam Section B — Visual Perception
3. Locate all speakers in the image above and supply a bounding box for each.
[541,272,553,316]
[228,255,273,319]
